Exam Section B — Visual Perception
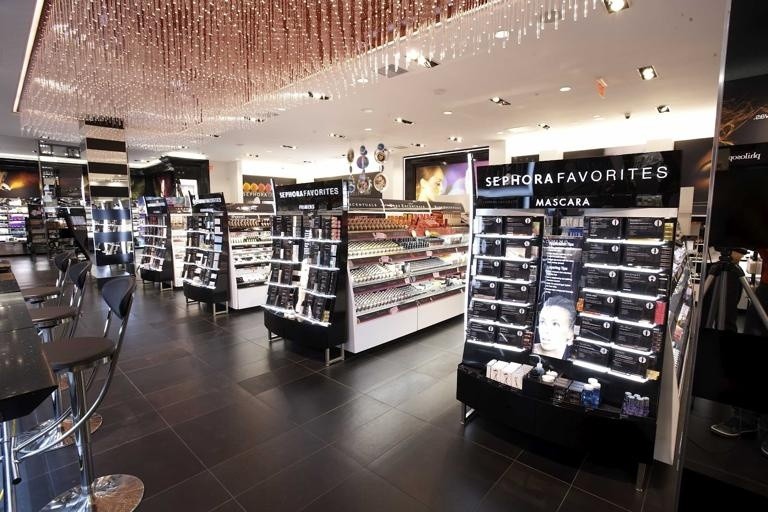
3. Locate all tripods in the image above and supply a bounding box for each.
[690,245,768,409]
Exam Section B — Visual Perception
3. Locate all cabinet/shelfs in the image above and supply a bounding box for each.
[266,203,469,344]
[0,203,45,256]
[187,214,273,302]
[457,162,706,449]
[131,211,189,276]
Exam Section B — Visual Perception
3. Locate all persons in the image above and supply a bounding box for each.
[708,247,768,456]
[532,296,575,359]
[415,165,445,201]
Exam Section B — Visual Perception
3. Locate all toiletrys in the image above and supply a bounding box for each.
[621,392,650,417]
[581,378,602,407]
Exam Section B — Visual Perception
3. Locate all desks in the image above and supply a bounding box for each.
[0,259,64,512]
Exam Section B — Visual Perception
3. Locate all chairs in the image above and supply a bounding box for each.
[18,252,105,456]
[19,247,75,395]
[8,276,151,512]
